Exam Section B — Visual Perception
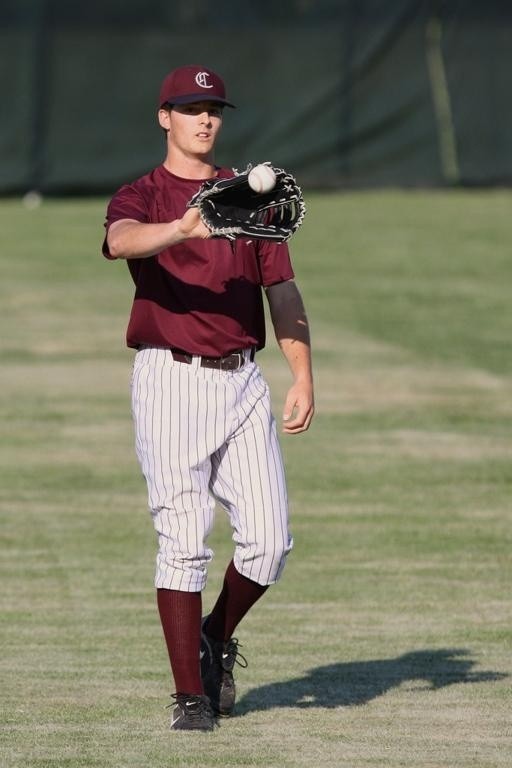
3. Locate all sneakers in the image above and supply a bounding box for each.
[197,614,248,719]
[165,693,217,732]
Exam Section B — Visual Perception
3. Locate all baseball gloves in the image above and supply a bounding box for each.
[186,162,306,245]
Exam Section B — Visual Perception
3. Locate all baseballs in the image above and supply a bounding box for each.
[248,164,276,193]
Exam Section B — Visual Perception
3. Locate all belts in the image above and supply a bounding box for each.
[171,347,257,372]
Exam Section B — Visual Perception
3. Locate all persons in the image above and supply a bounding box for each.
[102,68,315,730]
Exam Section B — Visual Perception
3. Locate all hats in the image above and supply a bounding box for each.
[160,64,238,109]
